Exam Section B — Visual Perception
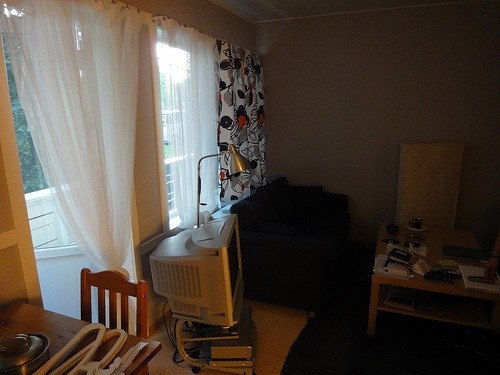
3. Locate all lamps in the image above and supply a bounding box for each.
[194,144,249,228]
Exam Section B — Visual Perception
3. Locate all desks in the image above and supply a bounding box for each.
[0,302,162,375]
[366,222,500,337]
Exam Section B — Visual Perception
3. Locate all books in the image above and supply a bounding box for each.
[458,264,500,295]
[383,246,411,278]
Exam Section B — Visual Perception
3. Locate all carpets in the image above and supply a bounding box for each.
[278,313,500,375]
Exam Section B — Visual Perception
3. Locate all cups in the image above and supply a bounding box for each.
[415,218,422,229]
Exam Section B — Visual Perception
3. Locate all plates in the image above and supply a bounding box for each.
[405,224,427,231]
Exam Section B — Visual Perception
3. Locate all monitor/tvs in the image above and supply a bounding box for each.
[149,214,244,325]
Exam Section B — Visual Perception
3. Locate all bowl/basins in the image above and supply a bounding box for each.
[0,333,51,375]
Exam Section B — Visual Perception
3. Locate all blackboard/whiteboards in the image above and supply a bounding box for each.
[396,142,465,231]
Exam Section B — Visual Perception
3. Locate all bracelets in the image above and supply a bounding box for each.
[490,255,500,259]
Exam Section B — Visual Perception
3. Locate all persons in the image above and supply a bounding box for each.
[484,228,500,281]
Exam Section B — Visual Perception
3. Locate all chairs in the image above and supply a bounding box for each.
[78,268,149,338]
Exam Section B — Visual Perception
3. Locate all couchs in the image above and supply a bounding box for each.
[230,177,350,318]
